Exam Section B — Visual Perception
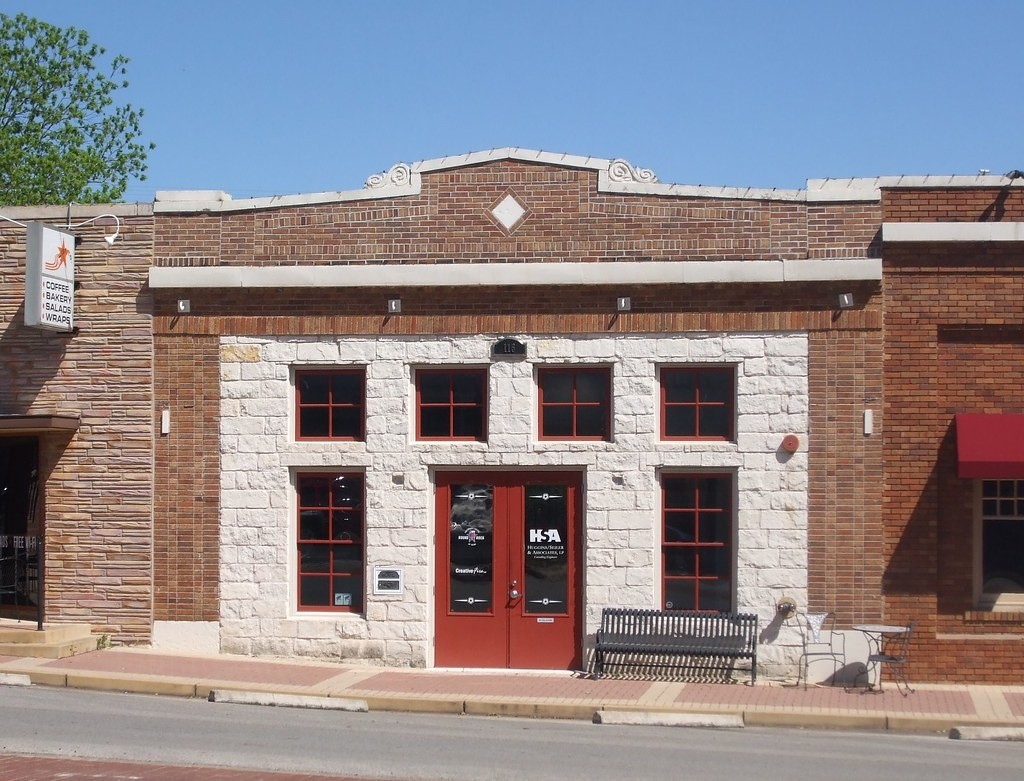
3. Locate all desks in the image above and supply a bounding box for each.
[853,626,915,698]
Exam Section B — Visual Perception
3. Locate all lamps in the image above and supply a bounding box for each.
[839,293,854,308]
[617,297,631,310]
[177,300,191,313]
[1006,170,1024,188]
[54,214,120,246]
[388,300,401,312]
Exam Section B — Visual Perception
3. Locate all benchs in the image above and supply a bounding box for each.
[591,607,758,685]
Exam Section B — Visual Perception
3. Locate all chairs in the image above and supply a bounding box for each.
[796,611,915,697]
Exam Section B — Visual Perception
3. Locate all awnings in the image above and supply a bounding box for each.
[955,413,1024,479]
